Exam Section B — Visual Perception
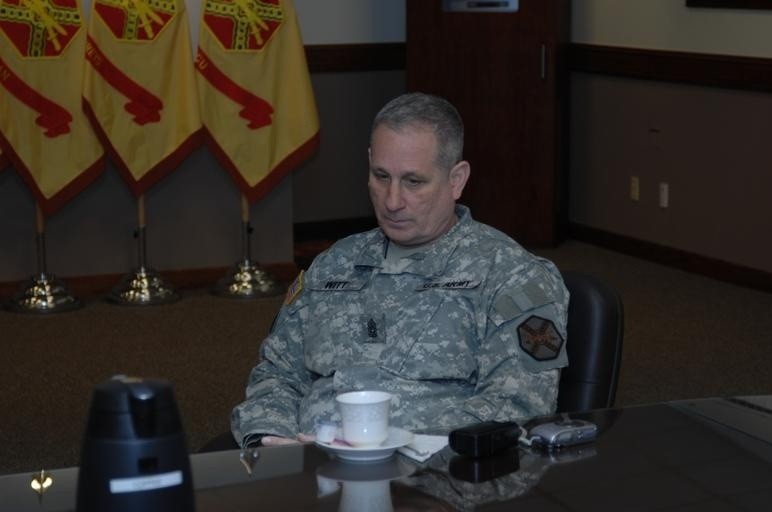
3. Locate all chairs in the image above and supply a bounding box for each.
[558,269,625,413]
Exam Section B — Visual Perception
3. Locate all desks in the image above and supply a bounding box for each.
[1,394,772,511]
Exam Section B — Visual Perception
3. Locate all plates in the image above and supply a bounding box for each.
[316,454,417,485]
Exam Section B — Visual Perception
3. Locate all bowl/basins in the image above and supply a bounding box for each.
[315,427,416,460]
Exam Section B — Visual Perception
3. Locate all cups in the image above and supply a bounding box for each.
[337,390,391,448]
[337,483,394,512]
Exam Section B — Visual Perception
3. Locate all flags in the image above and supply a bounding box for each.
[193,1,321,208]
[2,1,108,220]
[81,1,210,199]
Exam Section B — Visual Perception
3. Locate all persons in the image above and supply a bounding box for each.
[397,416,563,510]
[228,90,571,449]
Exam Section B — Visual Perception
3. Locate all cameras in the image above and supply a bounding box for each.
[530,419,598,450]
